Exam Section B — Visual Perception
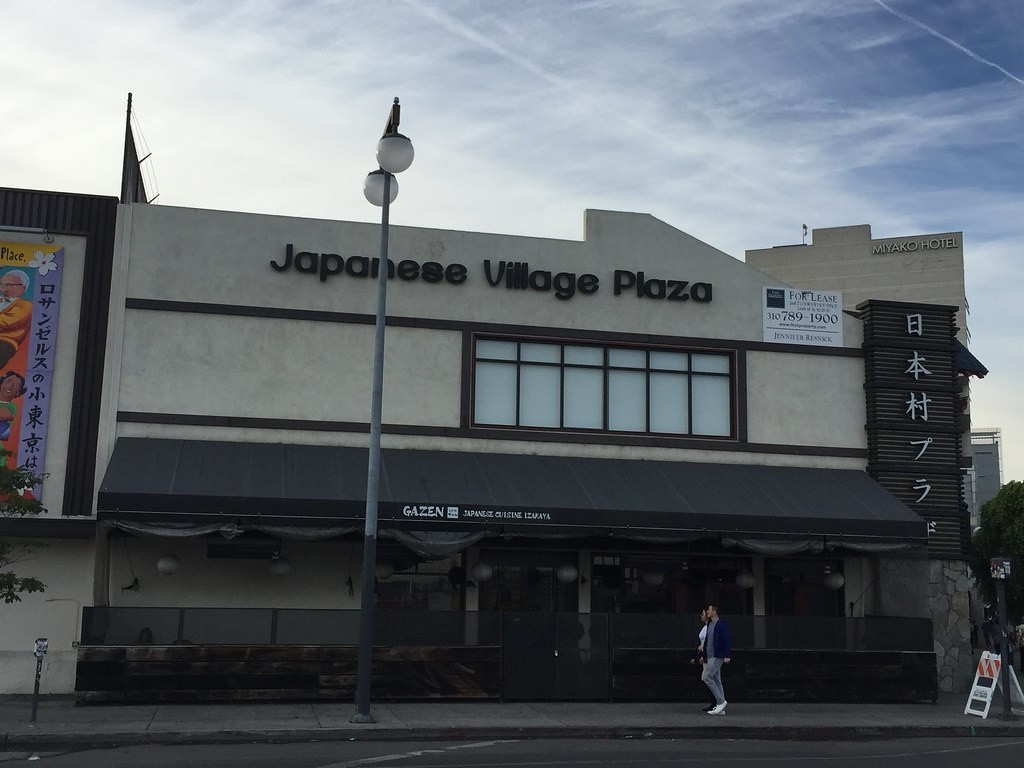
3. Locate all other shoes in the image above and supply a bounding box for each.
[702,704,716,713]
[711,701,727,714]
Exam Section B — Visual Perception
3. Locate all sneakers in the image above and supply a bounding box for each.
[707,707,725,715]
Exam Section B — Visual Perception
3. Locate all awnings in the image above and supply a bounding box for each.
[97,438,930,552]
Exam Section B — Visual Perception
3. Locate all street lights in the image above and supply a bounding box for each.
[350,98,414,726]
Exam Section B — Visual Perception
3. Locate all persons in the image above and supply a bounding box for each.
[699,601,732,715]
[690,607,717,711]
[970,615,1024,674]
